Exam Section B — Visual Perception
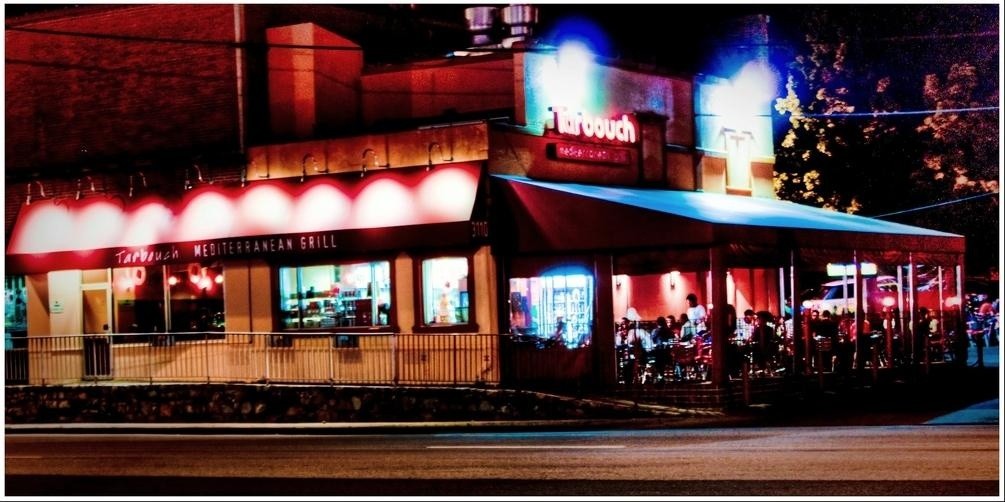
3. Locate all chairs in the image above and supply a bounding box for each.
[923,331,956,363]
[863,331,903,367]
[616,320,712,383]
[812,333,856,375]
[728,339,794,379]
